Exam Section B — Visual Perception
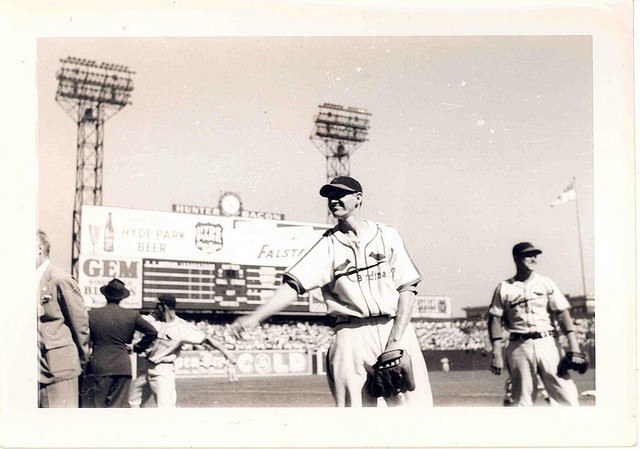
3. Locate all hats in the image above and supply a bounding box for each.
[153,294,176,309]
[512,241,543,258]
[100,278,130,299]
[320,175,363,198]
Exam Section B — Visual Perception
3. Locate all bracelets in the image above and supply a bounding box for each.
[490,337,502,342]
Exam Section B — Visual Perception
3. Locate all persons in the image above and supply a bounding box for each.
[183,321,334,352]
[228,175,434,407]
[126,293,236,408]
[487,241,589,406]
[37,229,90,407]
[87,277,158,408]
[411,318,493,353]
[554,317,595,347]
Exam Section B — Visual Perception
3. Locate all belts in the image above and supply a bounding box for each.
[510,330,555,340]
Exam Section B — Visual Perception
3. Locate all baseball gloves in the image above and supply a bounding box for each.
[368,349,415,398]
[558,351,590,376]
